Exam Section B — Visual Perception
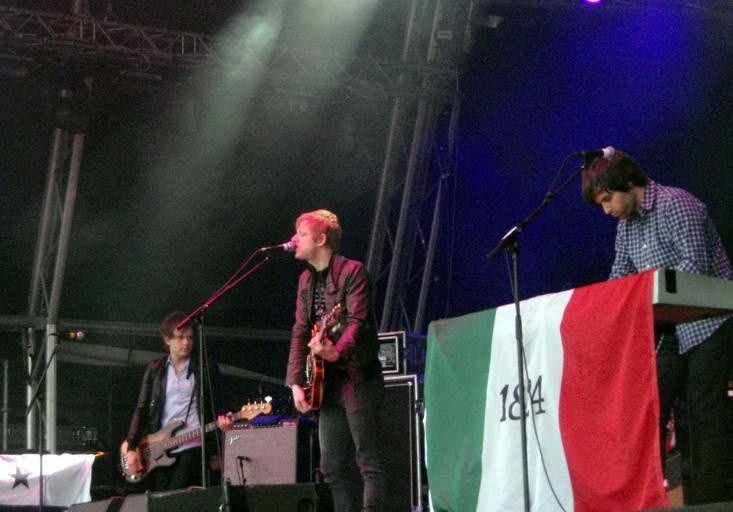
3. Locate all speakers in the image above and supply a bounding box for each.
[220,419,314,484]
[318,373,422,512]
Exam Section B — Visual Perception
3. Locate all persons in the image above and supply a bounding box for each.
[581,151,733,505]
[126,311,235,490]
[284,209,388,512]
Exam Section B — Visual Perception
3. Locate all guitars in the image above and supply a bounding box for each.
[121,396,272,482]
[302,304,341,411]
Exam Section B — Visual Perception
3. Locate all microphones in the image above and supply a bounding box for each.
[582,141,617,165]
[261,240,298,255]
[236,455,250,461]
[49,329,86,345]
